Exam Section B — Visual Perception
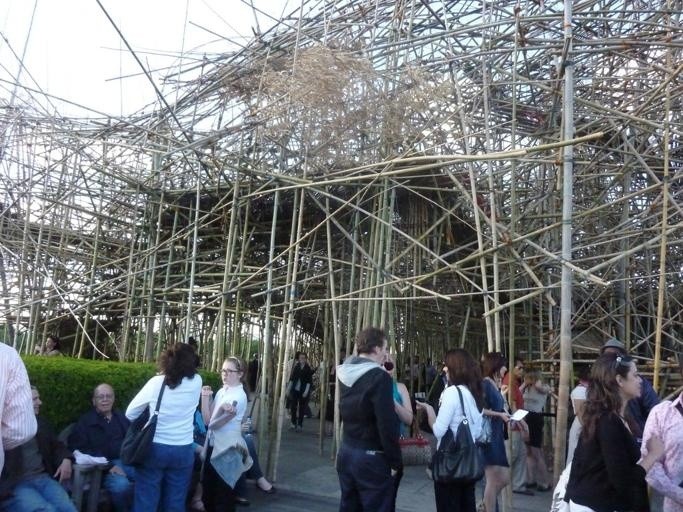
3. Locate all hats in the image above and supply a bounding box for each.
[598,338,628,355]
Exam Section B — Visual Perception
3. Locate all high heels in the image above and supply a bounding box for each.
[255,476,278,495]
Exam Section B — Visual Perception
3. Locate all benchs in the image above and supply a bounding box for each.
[46,419,134,512]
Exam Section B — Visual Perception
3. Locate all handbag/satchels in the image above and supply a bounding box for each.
[424,419,485,486]
[118,406,157,469]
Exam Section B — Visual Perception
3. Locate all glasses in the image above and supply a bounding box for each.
[515,365,525,370]
[219,368,241,376]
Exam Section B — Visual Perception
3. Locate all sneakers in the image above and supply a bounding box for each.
[286,413,333,440]
[512,477,553,497]
